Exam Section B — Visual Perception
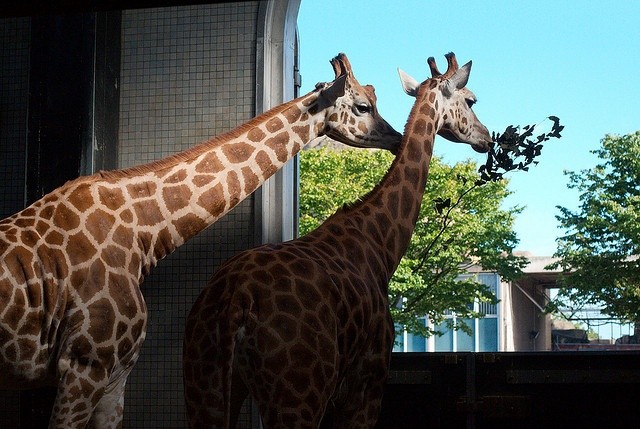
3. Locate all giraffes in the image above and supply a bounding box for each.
[1,52,405,428]
[183,51,496,429]
